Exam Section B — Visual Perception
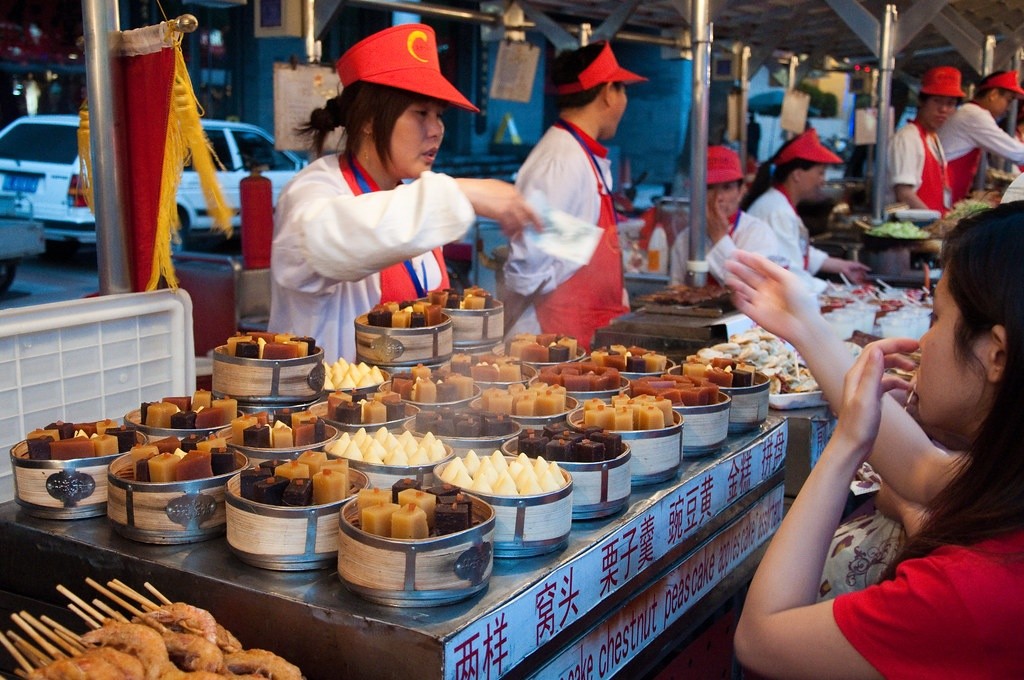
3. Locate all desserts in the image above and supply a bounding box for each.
[26,285,754,539]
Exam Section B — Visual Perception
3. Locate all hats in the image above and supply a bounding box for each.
[546,40,649,94]
[780,128,843,163]
[337,25,480,113]
[983,71,1023,99]
[921,67,966,97]
[707,146,744,183]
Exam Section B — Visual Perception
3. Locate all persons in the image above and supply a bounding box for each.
[879,66,967,222]
[741,130,872,285]
[872,477,936,540]
[668,146,791,288]
[263,22,547,366]
[939,70,1024,209]
[715,200,1024,680]
[497,38,650,348]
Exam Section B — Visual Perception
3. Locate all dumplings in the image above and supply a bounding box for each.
[695,325,822,393]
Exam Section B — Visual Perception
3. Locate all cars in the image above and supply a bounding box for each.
[744,84,918,181]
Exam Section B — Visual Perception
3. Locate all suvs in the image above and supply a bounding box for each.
[0,113,308,259]
